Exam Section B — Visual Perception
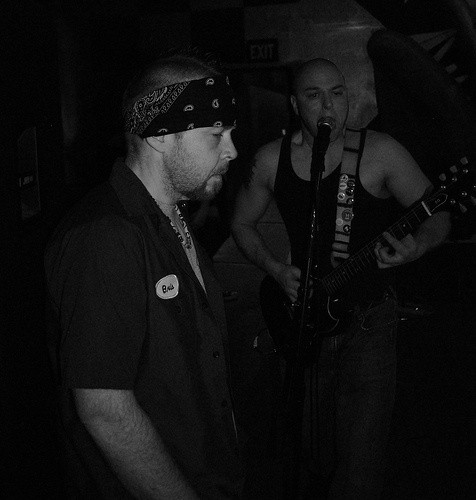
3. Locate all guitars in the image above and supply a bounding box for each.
[258,162,476,365]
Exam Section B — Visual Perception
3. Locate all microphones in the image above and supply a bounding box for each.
[312,115,337,169]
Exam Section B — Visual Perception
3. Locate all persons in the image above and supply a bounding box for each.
[231,56,452,500]
[42,56,247,499]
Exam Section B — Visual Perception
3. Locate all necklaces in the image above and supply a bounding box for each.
[162,203,192,249]
